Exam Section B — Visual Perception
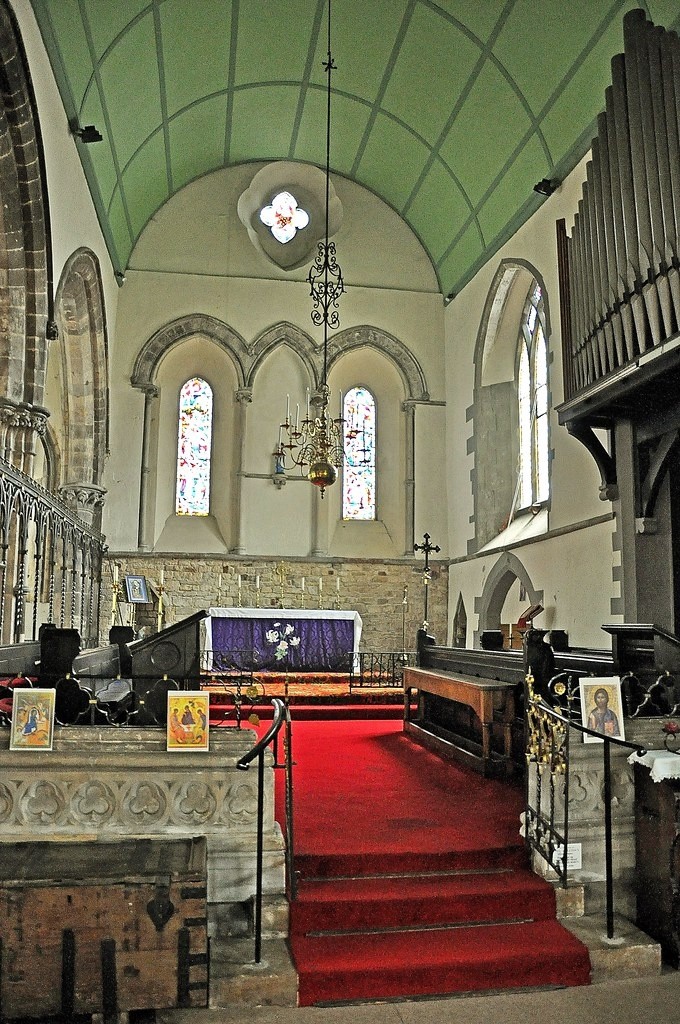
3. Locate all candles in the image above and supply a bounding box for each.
[335,578,339,590]
[319,578,322,590]
[219,574,221,587]
[159,570,163,584]
[237,574,241,587]
[301,577,304,590]
[255,576,259,588]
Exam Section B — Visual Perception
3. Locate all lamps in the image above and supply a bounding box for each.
[272,1,371,500]
[71,124,103,144]
[444,293,455,302]
[532,178,555,197]
[114,270,127,281]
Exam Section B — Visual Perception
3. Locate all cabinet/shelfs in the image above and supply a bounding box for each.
[627,750,680,971]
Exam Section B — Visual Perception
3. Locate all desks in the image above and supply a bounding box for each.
[203,607,363,674]
[401,665,517,779]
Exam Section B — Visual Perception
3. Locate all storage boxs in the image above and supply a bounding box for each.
[0,836,209,1024]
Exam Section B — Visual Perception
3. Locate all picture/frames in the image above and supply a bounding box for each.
[125,574,153,603]
[10,688,55,752]
[579,676,625,744]
[167,691,211,753]
